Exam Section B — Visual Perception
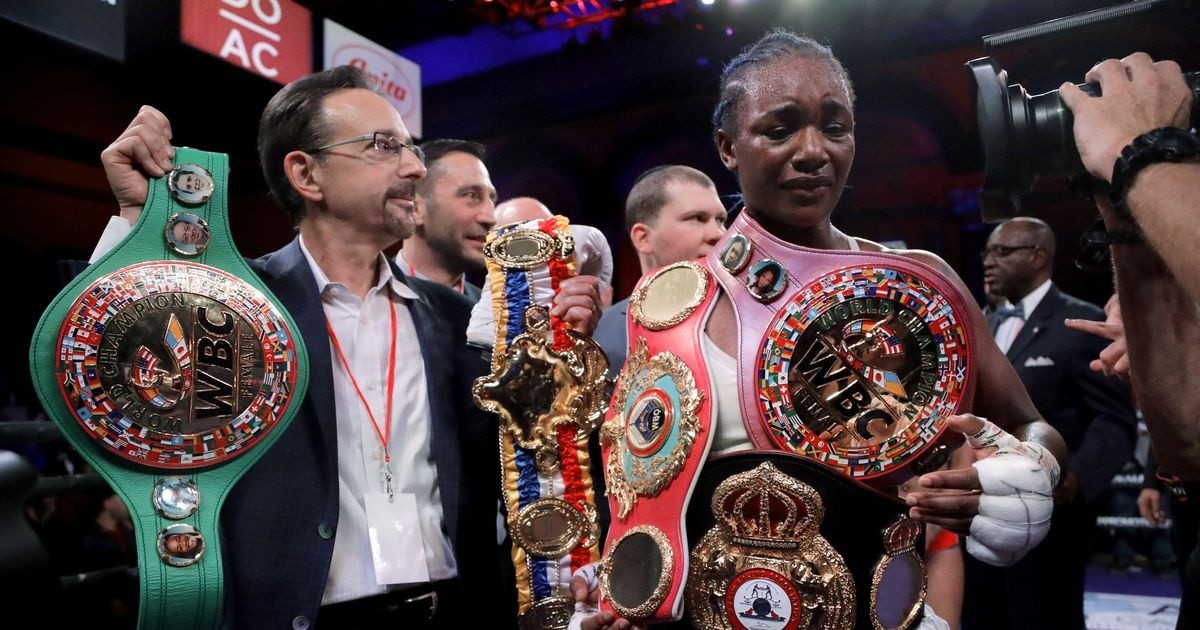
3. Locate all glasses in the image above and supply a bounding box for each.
[983,245,1040,256]
[304,132,425,167]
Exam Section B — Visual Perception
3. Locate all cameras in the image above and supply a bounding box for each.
[962,52,1200,206]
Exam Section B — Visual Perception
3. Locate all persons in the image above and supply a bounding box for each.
[90,479,137,568]
[1063,318,1131,381]
[88,65,497,630]
[985,217,1139,630]
[490,197,554,231]
[591,165,728,378]
[981,248,1015,318]
[1137,438,1200,630]
[591,31,1065,630]
[389,136,498,304]
[1059,52,1200,511]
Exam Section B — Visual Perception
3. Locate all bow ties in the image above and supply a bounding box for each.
[995,303,1024,321]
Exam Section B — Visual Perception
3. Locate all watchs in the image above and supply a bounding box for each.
[1111,126,1200,206]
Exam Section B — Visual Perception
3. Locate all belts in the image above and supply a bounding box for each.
[317,589,480,630]
